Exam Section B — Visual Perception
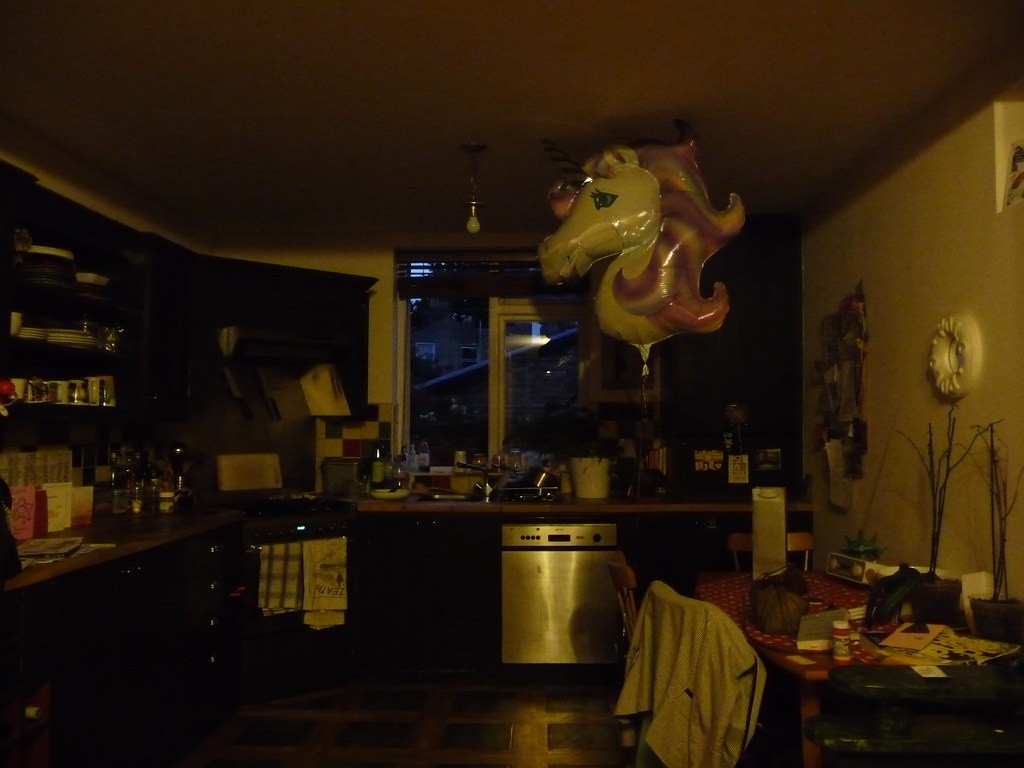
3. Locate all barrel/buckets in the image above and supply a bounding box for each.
[572,457,611,498]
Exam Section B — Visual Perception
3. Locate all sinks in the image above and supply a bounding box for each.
[416,492,479,503]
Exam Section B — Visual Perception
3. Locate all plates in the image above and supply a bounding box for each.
[371,489,409,499]
[11,310,98,347]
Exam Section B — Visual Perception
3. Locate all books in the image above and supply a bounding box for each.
[878,622,946,649]
[797,607,850,652]
[7,481,96,568]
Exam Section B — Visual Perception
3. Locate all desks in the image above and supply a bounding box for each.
[697,573,1024,768]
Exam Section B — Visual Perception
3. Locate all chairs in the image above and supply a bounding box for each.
[614,581,766,768]
[730,533,816,573]
[608,563,638,642]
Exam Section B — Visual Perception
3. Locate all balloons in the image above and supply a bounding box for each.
[535,120,748,367]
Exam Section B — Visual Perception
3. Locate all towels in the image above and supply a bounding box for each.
[261,537,346,632]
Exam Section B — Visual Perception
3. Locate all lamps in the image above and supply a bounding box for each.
[460,143,487,234]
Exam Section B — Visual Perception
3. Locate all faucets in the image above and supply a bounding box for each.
[456,461,494,503]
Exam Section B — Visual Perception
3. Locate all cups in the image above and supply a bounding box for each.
[455,451,466,472]
[509,449,521,472]
[69,380,88,404]
[28,380,48,402]
[84,377,100,404]
[49,380,69,403]
[492,455,505,470]
[98,375,114,404]
[9,378,26,399]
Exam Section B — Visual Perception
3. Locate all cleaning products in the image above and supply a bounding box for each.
[370,440,410,488]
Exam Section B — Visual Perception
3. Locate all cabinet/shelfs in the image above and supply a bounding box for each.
[0,161,155,424]
[183,520,243,758]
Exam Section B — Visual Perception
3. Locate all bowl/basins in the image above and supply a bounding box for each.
[526,467,554,489]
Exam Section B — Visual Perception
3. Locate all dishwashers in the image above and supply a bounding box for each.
[499,520,627,667]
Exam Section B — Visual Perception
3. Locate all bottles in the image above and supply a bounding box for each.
[384,452,393,487]
[419,442,430,471]
[394,454,408,488]
[111,447,191,519]
[832,621,850,660]
[372,448,384,488]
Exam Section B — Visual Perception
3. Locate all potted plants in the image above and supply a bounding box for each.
[969,424,1024,643]
[896,417,1003,627]
[503,416,625,501]
